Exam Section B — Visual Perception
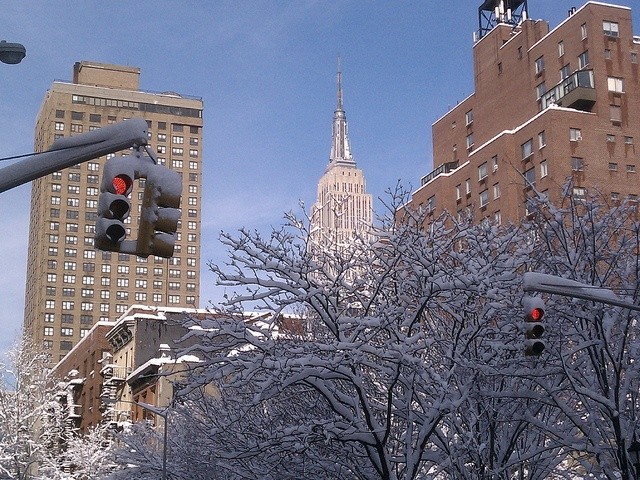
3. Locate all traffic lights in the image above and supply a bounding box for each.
[525,297,546,355]
[137,164,182,258]
[95,158,134,253]
